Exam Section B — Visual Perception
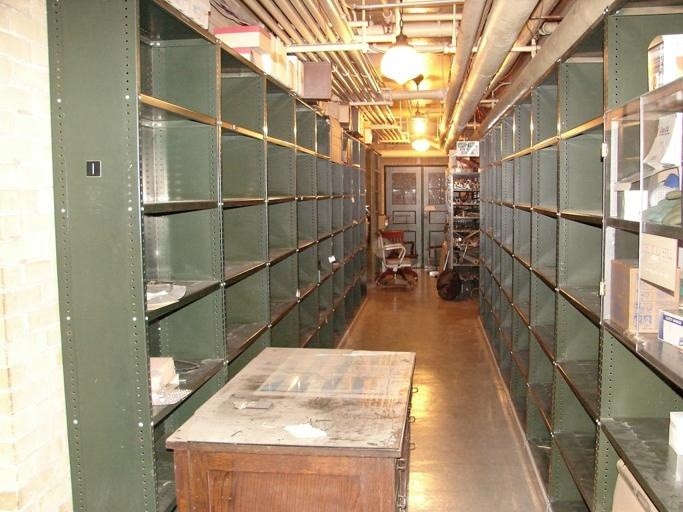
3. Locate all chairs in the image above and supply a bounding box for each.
[376,230,419,287]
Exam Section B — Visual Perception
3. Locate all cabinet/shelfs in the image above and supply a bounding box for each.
[167,347,420,511]
[479,3,680,511]
[49,2,379,512]
[445,149,478,301]
